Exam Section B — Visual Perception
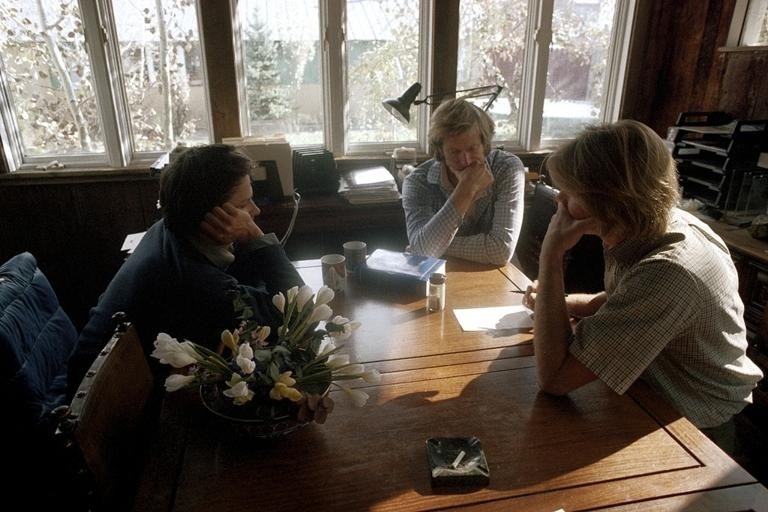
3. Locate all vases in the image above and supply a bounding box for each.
[200,348,331,441]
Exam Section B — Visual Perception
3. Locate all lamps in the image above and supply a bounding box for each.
[383,83,503,125]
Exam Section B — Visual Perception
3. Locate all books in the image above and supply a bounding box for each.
[358,247,447,298]
[292,145,402,209]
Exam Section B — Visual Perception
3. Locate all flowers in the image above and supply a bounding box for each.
[149,285,382,423]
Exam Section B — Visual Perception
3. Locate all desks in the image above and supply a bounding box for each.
[133,258,768,512]
[254,194,405,239]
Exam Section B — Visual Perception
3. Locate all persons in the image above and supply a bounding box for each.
[400,98,526,267]
[519,119,764,451]
[60,142,320,398]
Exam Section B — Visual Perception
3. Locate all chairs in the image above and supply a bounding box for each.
[51,310,163,512]
[0,252,79,428]
[516,183,576,281]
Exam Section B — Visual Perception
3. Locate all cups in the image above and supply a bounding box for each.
[343,241,366,276]
[321,254,346,294]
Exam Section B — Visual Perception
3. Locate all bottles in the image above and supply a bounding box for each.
[427,272,446,312]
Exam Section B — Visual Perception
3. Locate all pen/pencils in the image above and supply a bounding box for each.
[510,291,525,294]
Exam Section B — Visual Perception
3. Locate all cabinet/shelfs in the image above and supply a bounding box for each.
[669,111,768,221]
